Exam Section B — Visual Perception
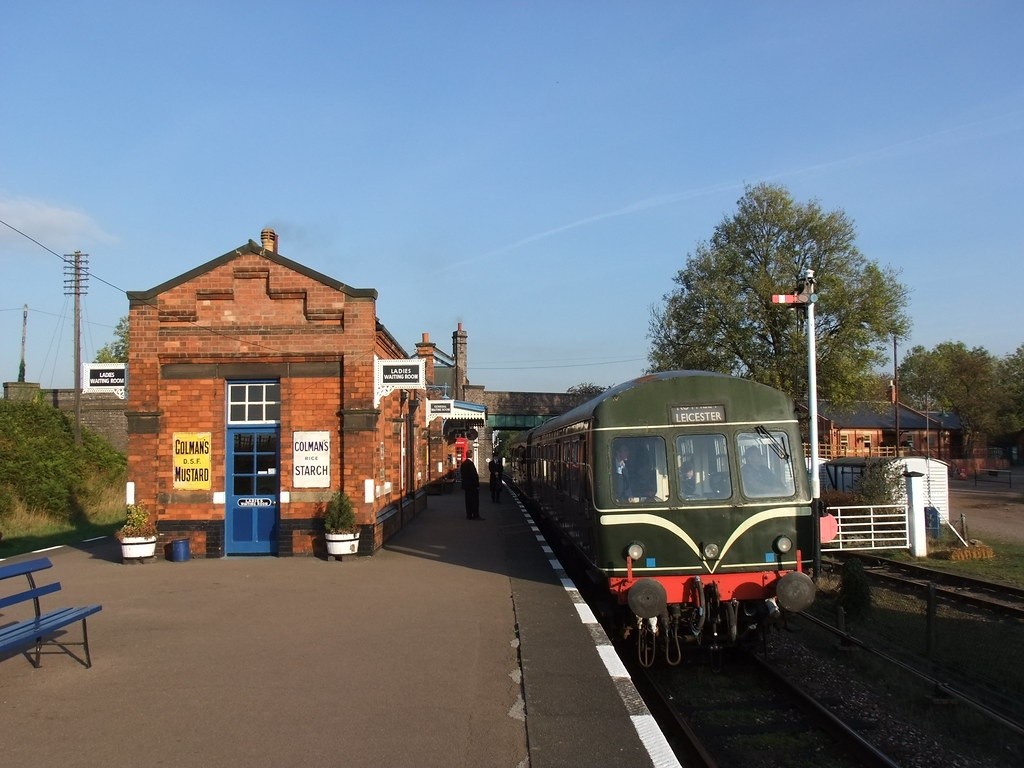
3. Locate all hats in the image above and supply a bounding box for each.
[492,452,499,456]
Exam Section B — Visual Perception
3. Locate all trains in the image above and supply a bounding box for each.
[494,369,817,676]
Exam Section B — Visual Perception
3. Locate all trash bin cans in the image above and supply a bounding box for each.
[171,540,190,562]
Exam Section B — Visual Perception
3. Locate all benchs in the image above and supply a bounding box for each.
[0,557,104,669]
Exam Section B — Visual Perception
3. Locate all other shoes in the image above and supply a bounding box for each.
[473,513,485,520]
[466,514,473,519]
[492,500,501,503]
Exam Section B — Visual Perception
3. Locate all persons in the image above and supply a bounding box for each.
[612,444,632,504]
[460,449,486,522]
[740,445,790,496]
[679,462,695,494]
[488,451,503,502]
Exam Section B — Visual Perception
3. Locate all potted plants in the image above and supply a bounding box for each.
[115,498,160,559]
[323,490,363,556]
